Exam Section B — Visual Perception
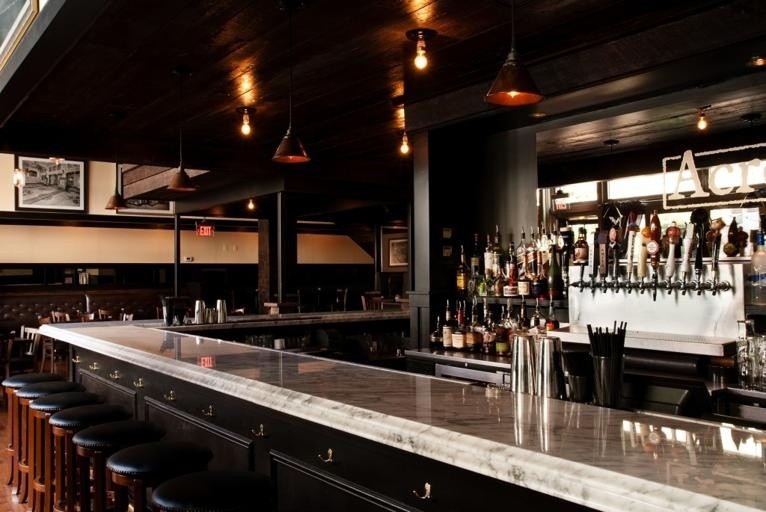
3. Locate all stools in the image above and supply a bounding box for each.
[2,373,278,512]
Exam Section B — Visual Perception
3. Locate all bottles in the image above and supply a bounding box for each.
[430,219,588,357]
[239,332,308,353]
[750,231,766,306]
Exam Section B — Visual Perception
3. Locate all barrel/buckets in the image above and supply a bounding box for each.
[162,295,189,326]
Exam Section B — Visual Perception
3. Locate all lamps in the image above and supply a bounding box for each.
[237,107,256,135]
[696,106,710,130]
[167,72,197,192]
[486,0,544,108]
[406,28,437,69]
[398,130,411,156]
[272,11,312,164]
[106,110,128,209]
[247,198,255,210]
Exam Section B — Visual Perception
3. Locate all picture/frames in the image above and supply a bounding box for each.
[381,233,410,274]
[15,153,89,213]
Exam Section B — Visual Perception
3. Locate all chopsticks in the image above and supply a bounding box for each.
[584,320,628,356]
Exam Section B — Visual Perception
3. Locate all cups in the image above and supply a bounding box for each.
[510,390,564,460]
[593,406,626,467]
[562,373,593,405]
[507,333,566,399]
[591,353,625,410]
[194,299,228,326]
[562,400,591,433]
[737,318,766,392]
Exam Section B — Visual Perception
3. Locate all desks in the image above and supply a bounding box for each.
[255,288,322,314]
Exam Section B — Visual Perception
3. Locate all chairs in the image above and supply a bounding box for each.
[361,296,385,310]
[329,288,348,310]
[0,307,163,405]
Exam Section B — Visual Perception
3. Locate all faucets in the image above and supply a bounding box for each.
[574,236,731,304]
